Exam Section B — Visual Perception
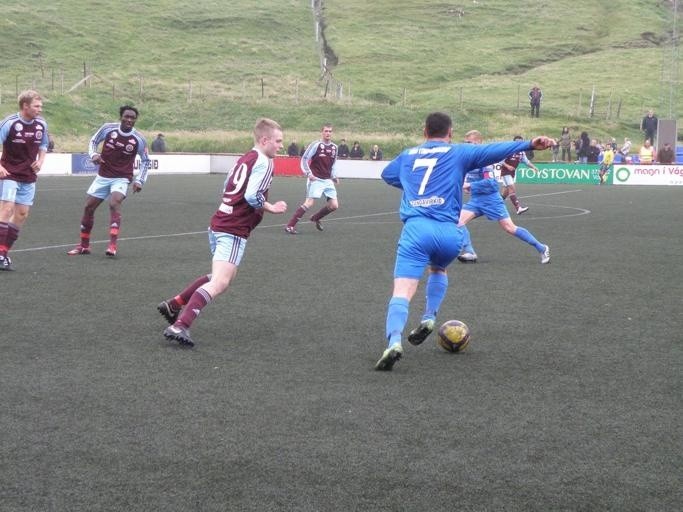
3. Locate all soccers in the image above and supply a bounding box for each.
[437,319,474,353]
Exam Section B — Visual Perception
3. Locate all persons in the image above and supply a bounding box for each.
[147,133,166,153]
[287,124,339,235]
[371,146,382,160]
[489,136,541,215]
[66,106,150,256]
[350,141,366,160]
[0,91,48,271]
[528,84,542,118]
[287,139,300,156]
[45,135,56,154]
[157,119,287,346]
[599,146,615,183]
[550,138,560,161]
[620,138,632,155]
[560,126,572,161]
[587,138,600,162]
[453,129,551,264]
[375,111,558,371]
[639,141,655,164]
[577,132,589,164]
[337,138,349,158]
[656,145,676,164]
[639,108,658,145]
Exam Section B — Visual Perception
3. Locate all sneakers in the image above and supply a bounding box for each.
[541,245,550,264]
[163,325,195,348]
[284,227,301,234]
[6,257,16,271]
[408,320,434,345]
[517,207,529,215]
[106,245,116,256]
[157,300,179,326]
[310,215,323,231]
[0,255,9,269]
[67,245,91,255]
[457,253,478,262]
[375,343,402,371]
